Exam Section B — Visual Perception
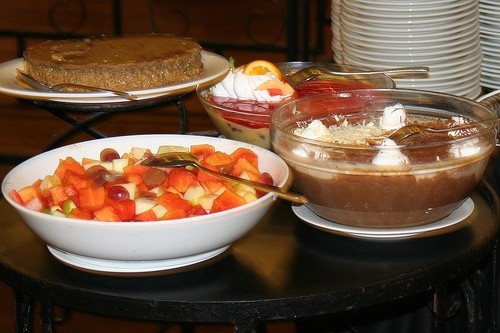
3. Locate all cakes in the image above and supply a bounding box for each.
[20,33,205,92]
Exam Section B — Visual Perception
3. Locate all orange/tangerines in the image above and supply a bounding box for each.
[244,60,282,80]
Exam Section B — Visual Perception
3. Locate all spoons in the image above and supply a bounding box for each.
[16,66,139,101]
[140,153,309,205]
[285,66,429,90]
[389,114,499,146]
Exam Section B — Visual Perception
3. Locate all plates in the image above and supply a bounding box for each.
[330,0,500,102]
[291,197,474,241]
[0,50,230,111]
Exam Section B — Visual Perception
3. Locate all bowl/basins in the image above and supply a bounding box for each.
[196,61,395,151]
[268,89,499,228]
[1,133,294,277]
